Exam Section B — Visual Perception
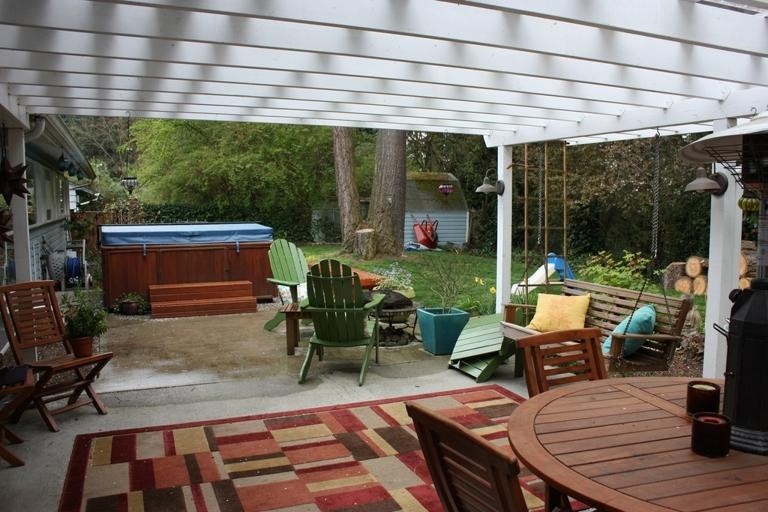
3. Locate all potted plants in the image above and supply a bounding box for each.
[113,291,149,315]
[411,254,469,356]
[59,285,109,358]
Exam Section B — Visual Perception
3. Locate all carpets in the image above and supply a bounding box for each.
[58,384,585,512]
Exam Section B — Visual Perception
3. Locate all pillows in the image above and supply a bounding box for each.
[602,303,656,356]
[524,293,591,334]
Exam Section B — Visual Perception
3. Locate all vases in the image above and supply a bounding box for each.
[396,288,416,302]
[371,307,416,349]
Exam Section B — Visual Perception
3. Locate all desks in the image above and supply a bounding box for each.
[507,376,767,512]
[0,368,39,466]
[305,260,381,302]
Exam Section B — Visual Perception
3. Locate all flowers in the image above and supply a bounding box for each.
[375,263,413,290]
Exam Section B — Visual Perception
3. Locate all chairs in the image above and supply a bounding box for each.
[298,261,381,387]
[516,329,608,398]
[404,399,528,512]
[1,280,113,432]
[264,238,309,331]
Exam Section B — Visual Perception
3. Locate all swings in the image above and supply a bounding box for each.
[499,132,692,373]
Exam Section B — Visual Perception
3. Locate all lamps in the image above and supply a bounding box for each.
[439,184,454,197]
[475,167,505,196]
[684,167,728,201]
[57,154,84,181]
[738,187,762,218]
[123,176,138,194]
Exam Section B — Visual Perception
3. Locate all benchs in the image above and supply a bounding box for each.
[501,279,692,372]
[278,303,311,355]
